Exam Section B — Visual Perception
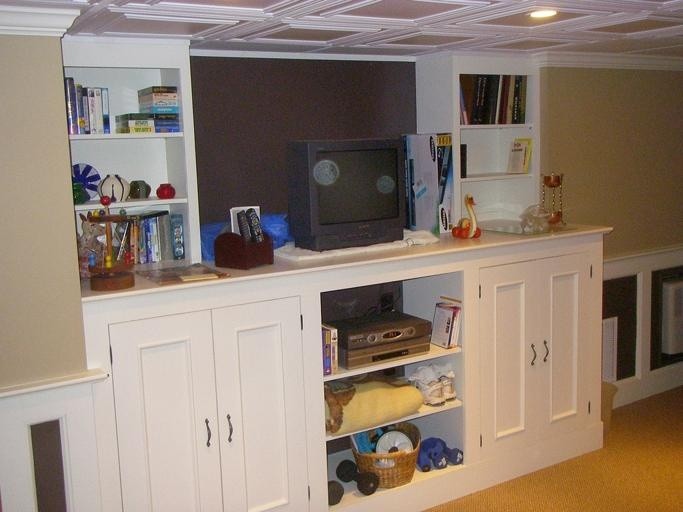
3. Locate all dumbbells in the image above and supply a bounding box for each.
[337,459,378,495]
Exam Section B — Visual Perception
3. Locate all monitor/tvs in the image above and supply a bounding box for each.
[287,135,406,252]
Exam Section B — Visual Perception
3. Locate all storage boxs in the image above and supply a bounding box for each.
[215,226,273,270]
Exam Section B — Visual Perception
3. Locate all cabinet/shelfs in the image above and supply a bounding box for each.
[414,46,541,232]
[398,231,601,496]
[77,260,312,512]
[59,31,201,278]
[314,249,468,508]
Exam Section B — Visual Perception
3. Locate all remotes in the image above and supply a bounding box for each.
[245,208,266,244]
[237,210,252,244]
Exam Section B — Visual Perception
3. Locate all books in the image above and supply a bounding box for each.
[429,295,460,348]
[112,210,184,265]
[64,77,180,134]
[458,74,525,124]
[320,327,331,376]
[504,137,534,175]
[322,317,338,374]
